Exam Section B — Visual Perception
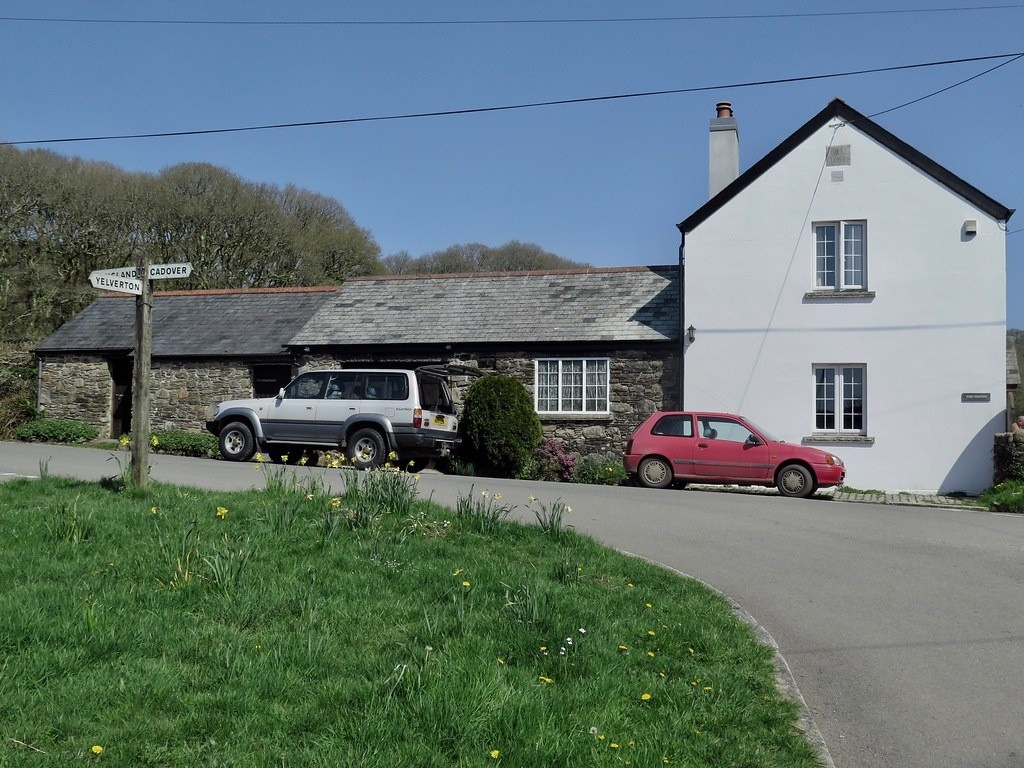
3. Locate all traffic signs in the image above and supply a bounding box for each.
[148,263,194,279]
[88,267,142,295]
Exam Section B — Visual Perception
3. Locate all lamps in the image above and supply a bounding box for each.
[687,323,696,342]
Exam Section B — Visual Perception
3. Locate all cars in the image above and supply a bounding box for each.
[624,411,847,498]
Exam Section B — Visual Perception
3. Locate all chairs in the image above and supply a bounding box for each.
[366,386,384,399]
[326,383,346,399]
[704,424,712,439]
[709,429,718,439]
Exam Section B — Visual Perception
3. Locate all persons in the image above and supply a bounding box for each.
[1012,416,1024,430]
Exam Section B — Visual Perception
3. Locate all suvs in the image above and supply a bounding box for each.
[205,363,486,470]
